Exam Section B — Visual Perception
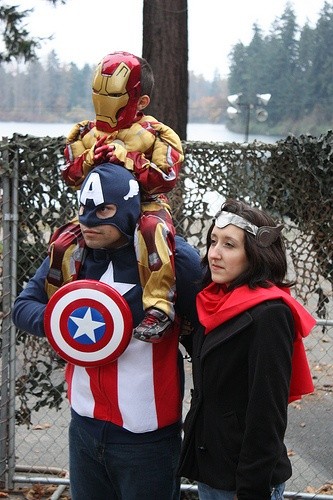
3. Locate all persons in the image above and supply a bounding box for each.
[12,163,203,500]
[178,200,317,500]
[44,52,185,343]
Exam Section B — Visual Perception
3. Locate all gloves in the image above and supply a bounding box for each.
[93,134,124,165]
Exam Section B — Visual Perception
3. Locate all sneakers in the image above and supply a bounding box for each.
[134,308,174,343]
